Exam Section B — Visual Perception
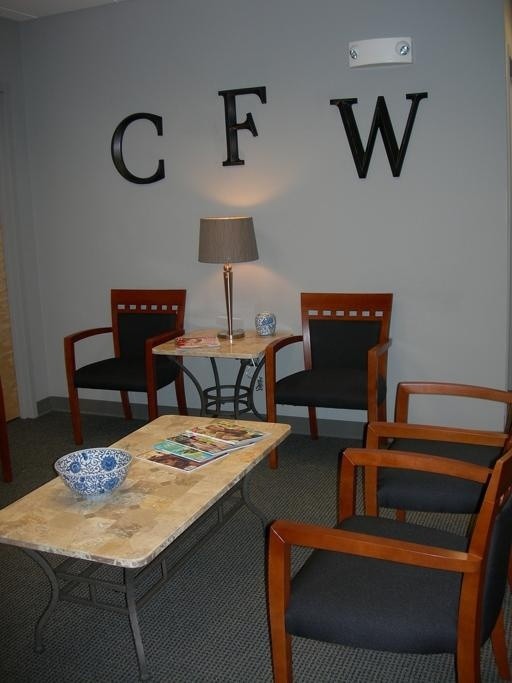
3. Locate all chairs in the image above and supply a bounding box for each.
[265,292,393,467]
[268,381,512,678]
[64,289,187,445]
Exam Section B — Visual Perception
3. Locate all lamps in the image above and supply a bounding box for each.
[197,215,259,340]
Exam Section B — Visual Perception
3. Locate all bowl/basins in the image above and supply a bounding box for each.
[54,446,133,500]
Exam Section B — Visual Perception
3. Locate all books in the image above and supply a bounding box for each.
[152,439,231,462]
[134,448,228,473]
[165,430,255,454]
[175,334,222,348]
[186,416,269,445]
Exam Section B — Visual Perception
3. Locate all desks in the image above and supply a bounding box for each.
[152,331,289,420]
